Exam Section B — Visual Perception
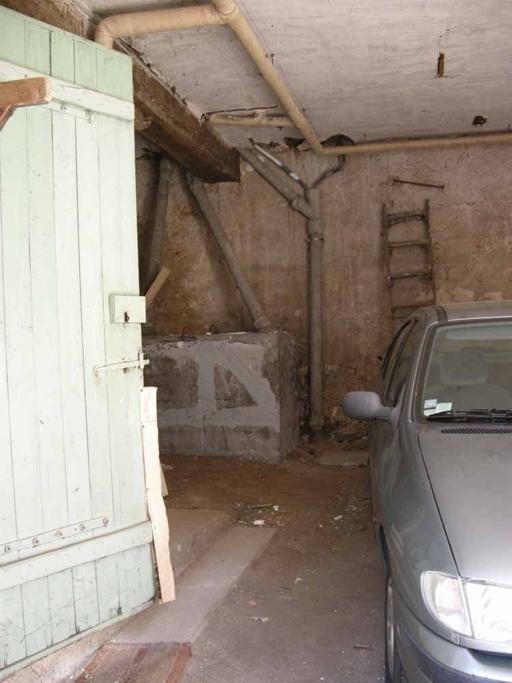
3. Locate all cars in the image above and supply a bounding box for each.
[342,301,512,682]
